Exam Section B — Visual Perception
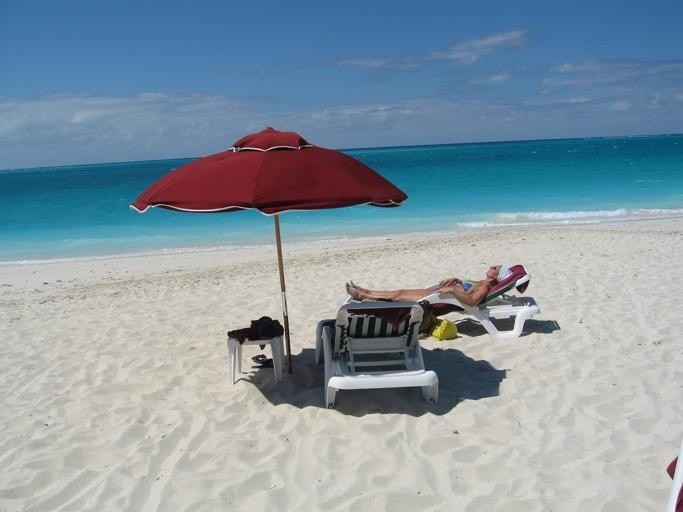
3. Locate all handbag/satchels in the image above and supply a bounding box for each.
[429,318,457,341]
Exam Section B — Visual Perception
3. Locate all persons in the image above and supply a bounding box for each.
[343,259,512,308]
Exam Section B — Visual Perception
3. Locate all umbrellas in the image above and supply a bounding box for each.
[128,122,408,373]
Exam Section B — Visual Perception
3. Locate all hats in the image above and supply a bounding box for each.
[496,264,513,282]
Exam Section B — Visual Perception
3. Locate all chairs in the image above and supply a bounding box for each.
[315,263,541,410]
